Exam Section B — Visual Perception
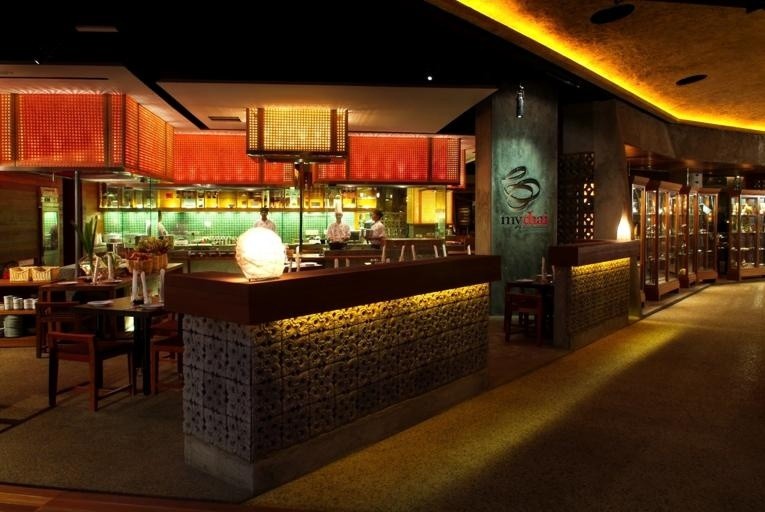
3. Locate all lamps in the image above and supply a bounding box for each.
[515,88,525,118]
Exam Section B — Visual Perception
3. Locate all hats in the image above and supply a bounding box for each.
[334,199,342,213]
[260,208,268,213]
[373,209,383,218]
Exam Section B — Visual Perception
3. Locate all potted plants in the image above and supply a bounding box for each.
[71,215,102,276]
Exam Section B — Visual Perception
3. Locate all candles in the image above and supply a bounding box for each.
[551,264,555,273]
[92,256,100,283]
[541,256,545,279]
[131,268,165,304]
[107,255,114,279]
[346,257,350,267]
[334,258,339,268]
[288,246,301,272]
[381,243,471,264]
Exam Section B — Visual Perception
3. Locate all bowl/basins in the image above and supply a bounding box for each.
[0,293,38,311]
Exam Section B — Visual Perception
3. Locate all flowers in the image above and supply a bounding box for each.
[134,236,171,256]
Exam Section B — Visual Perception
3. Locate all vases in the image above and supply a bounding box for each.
[152,253,168,272]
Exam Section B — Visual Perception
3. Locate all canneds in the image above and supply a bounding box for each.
[3,295,34,310]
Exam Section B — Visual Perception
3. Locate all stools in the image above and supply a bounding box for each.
[149,331,185,396]
[505,292,543,346]
[35,301,80,358]
[47,327,138,412]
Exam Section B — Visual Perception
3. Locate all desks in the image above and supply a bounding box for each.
[504,278,553,341]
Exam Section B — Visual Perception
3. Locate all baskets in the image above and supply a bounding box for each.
[32,267,59,280]
[9,266,32,281]
[147,253,168,271]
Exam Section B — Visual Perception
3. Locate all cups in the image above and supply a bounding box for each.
[153,253,168,272]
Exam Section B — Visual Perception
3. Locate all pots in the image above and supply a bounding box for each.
[3,314,24,338]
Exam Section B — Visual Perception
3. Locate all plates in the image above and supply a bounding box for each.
[86,301,114,307]
[140,303,165,310]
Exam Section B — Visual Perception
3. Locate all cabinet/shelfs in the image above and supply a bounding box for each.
[1,277,49,349]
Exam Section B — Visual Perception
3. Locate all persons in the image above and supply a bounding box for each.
[371,208,386,244]
[147,210,168,235]
[254,208,276,233]
[326,210,351,240]
